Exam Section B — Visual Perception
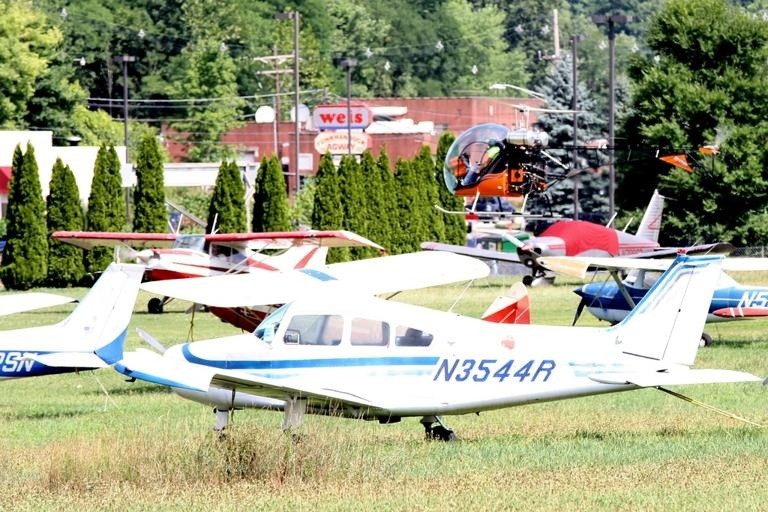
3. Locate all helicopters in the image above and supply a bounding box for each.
[423,94,728,225]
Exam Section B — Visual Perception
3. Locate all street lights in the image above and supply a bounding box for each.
[488,82,545,104]
[114,55,139,219]
[593,10,633,228]
[341,60,358,155]
[54,134,81,143]
[567,31,587,221]
[276,11,301,226]
[72,56,85,66]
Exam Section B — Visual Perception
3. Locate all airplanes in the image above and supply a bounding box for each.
[537,255,767,346]
[0,241,148,382]
[51,223,531,361]
[419,189,733,293]
[111,252,767,459]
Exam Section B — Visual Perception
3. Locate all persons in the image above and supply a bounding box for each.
[461,139,501,186]
